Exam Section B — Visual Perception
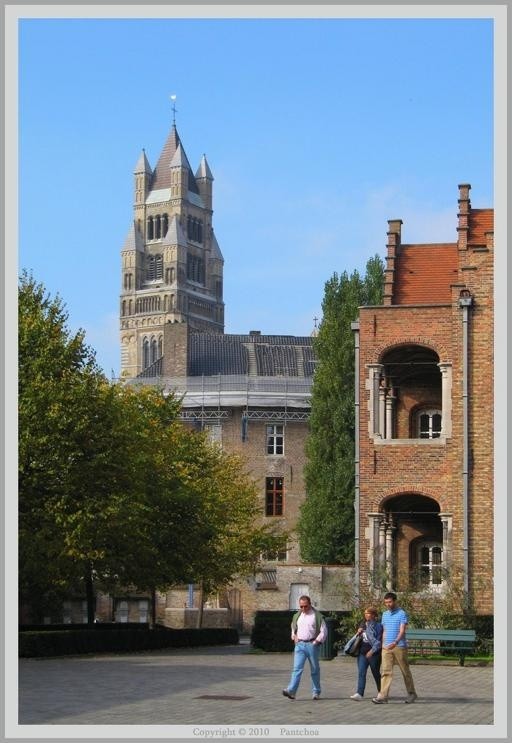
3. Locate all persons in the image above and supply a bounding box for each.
[349,605,384,702]
[282,595,330,701]
[371,592,418,704]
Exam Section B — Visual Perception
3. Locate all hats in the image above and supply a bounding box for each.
[364,607,378,617]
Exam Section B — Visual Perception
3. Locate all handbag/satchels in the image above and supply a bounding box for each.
[344,633,361,657]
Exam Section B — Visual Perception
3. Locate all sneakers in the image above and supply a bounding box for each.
[405,694,417,703]
[372,698,388,704]
[283,689,295,699]
[312,695,319,699]
[350,693,362,700]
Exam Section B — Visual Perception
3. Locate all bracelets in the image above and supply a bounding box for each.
[394,639,398,644]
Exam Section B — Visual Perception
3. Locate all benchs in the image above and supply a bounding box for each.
[398,627,480,665]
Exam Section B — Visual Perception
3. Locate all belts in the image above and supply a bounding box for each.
[298,638,314,642]
